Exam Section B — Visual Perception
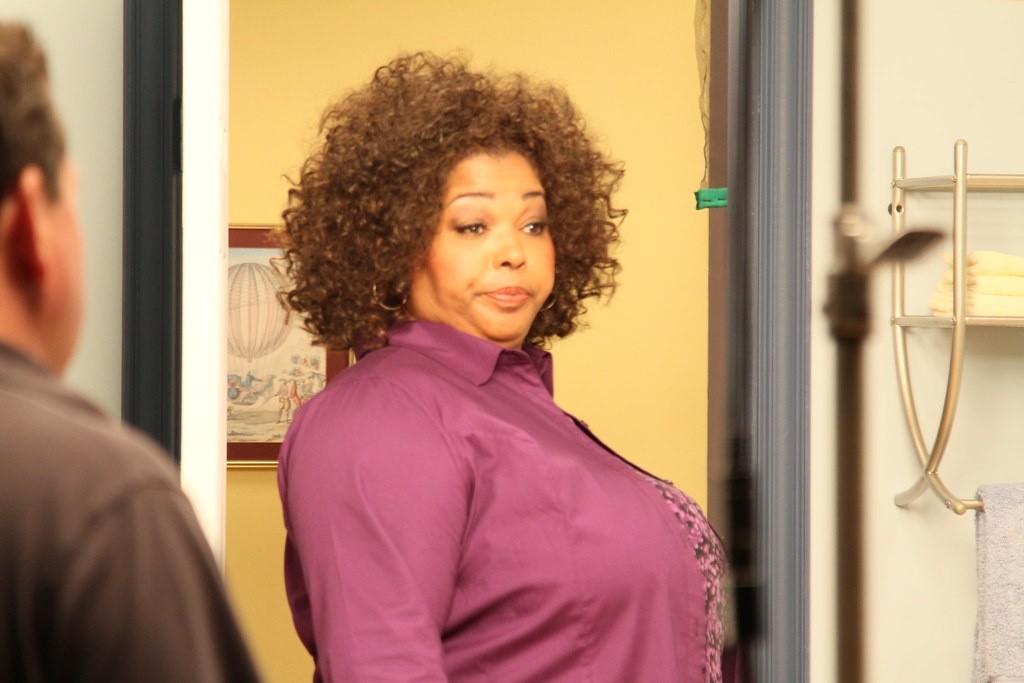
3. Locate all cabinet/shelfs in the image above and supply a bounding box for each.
[890,139,1024,517]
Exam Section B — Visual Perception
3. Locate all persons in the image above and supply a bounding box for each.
[277,51,726,683]
[0,20,263,683]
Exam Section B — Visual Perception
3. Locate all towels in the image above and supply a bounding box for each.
[930,250,1024,316]
[971,481,1024,683]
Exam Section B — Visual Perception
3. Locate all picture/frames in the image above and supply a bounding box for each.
[227,223,354,470]
